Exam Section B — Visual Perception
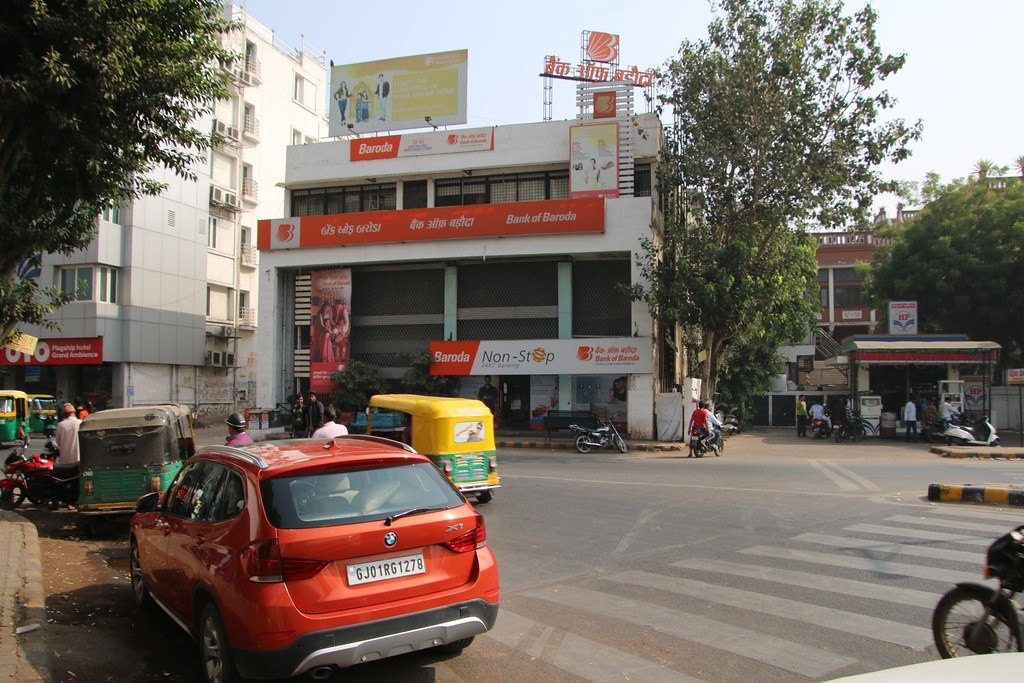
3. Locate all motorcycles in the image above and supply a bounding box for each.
[0,433,81,510]
[811,403,833,439]
[365,394,501,503]
[0,390,59,446]
[925,410,979,443]
[570,413,627,453]
[75,404,196,535]
[690,423,724,458]
[833,411,866,442]
[932,523,1024,659]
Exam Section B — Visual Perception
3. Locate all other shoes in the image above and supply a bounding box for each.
[68,504,75,510]
[52,501,62,508]
[700,441,706,447]
[904,438,909,441]
[687,454,692,458]
[803,434,806,436]
[913,439,918,442]
[798,435,801,436]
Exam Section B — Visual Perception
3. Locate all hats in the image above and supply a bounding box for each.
[63,403,76,413]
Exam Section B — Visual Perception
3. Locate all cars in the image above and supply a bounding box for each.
[130,434,500,683]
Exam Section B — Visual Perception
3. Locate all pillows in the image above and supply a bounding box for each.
[351,478,402,513]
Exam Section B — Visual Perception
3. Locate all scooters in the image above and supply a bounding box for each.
[944,412,1001,446]
[724,401,742,436]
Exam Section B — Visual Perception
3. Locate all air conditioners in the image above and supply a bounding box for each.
[225,193,236,207]
[210,186,223,204]
[228,127,239,141]
[212,119,226,137]
[206,350,236,367]
[220,59,252,85]
[222,327,235,337]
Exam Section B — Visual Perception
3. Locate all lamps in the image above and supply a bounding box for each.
[425,117,438,129]
[348,124,359,135]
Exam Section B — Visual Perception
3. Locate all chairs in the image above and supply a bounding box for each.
[320,474,359,504]
[317,496,349,512]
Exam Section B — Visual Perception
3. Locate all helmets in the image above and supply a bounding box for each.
[225,413,246,427]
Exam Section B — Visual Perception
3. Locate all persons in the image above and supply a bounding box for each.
[52,402,83,510]
[289,391,341,438]
[797,394,809,437]
[583,158,600,184]
[0,400,11,414]
[609,376,627,404]
[74,396,97,420]
[312,408,348,438]
[375,74,390,120]
[43,402,55,410]
[402,414,411,443]
[225,412,253,446]
[920,398,938,431]
[355,90,369,123]
[904,397,920,442]
[839,399,854,433]
[333,81,352,126]
[940,397,961,424]
[57,391,66,420]
[317,298,349,362]
[550,377,559,409]
[687,401,722,458]
[477,375,498,414]
[809,399,832,432]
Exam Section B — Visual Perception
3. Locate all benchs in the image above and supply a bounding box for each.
[322,412,404,434]
[543,410,600,441]
